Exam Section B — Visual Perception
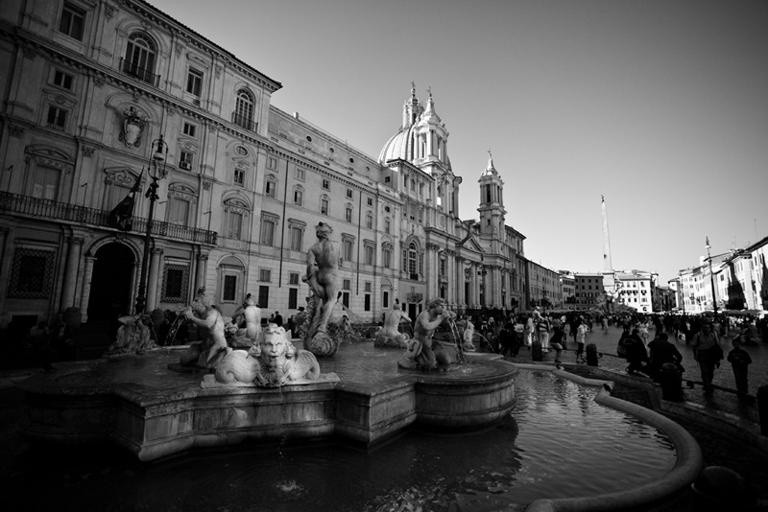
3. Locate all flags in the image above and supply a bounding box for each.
[106,169,143,231]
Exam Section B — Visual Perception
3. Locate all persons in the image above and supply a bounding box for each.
[383,303,411,344]
[412,299,457,368]
[306,226,339,339]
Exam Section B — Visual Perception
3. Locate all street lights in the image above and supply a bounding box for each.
[134,131,171,314]
[705,235,719,323]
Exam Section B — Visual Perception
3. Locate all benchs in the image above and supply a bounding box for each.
[600,367,686,421]
